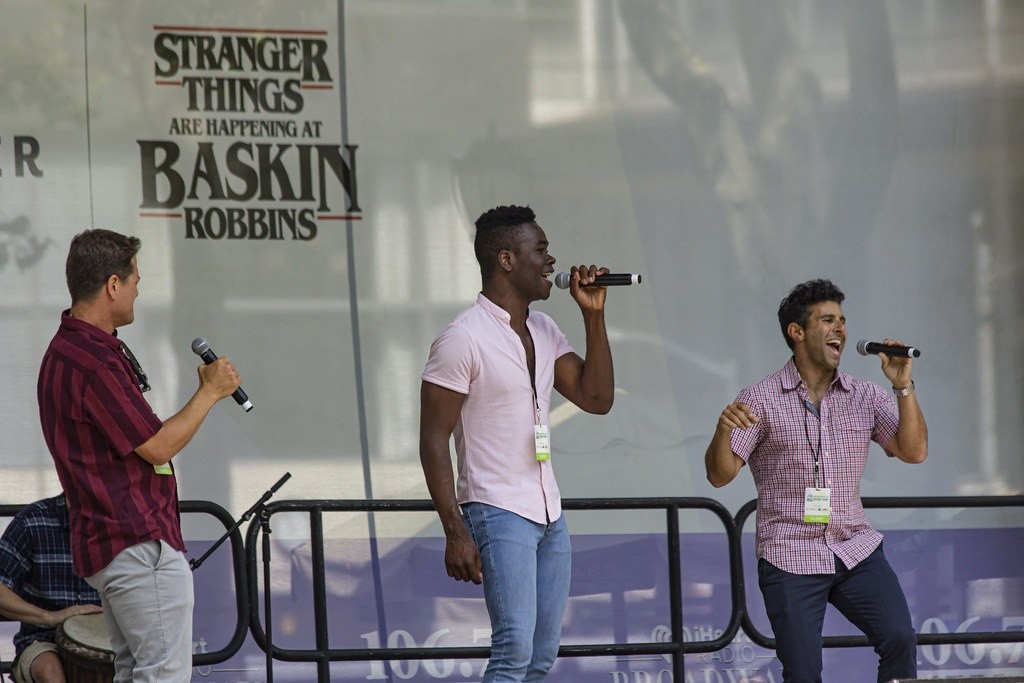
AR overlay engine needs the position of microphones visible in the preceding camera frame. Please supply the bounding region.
[555,272,642,290]
[857,339,920,359]
[191,338,254,413]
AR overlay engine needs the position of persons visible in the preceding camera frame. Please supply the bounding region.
[419,206,615,683]
[0,490,107,683]
[704,279,929,683]
[38,228,242,683]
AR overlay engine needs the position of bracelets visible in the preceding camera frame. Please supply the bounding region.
[892,379,915,397]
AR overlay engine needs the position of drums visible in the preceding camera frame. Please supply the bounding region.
[54,612,116,683]
